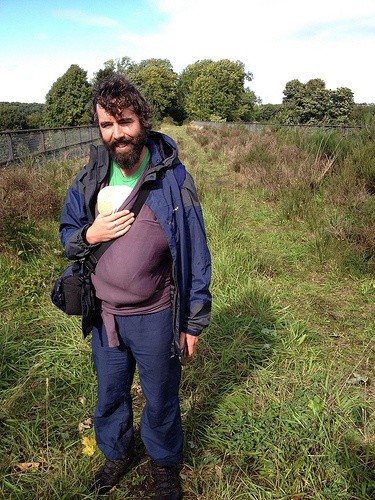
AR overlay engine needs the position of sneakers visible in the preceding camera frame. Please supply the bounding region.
[147,462,181,500]
[88,455,136,493]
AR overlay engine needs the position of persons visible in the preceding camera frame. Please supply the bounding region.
[55,76,215,500]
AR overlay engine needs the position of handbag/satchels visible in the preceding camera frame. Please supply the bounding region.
[48,260,84,314]
